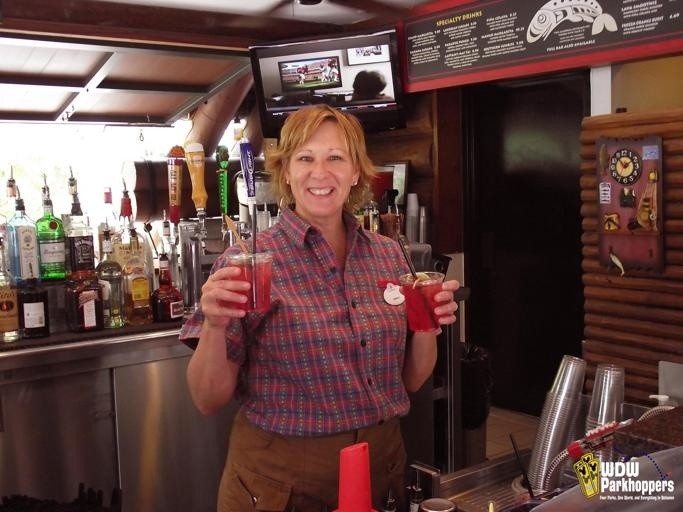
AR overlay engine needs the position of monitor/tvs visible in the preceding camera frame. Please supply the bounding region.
[248,26,407,139]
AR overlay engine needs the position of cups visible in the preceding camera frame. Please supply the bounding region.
[399,273,446,334]
[224,252,273,312]
[404,192,428,243]
[526,356,627,493]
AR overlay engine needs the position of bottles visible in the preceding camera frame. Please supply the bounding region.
[0,167,189,343]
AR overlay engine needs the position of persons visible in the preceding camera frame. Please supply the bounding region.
[316,58,339,83]
[173,102,463,512]
[295,65,308,85]
